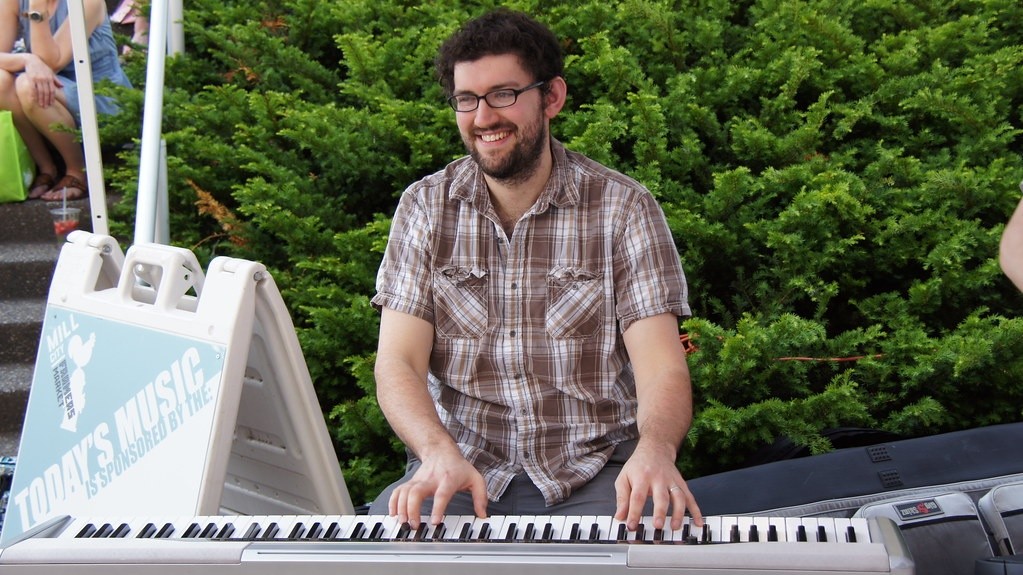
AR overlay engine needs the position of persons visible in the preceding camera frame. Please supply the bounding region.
[367,11,706,531]
[121,0,149,55]
[0,0,134,200]
[999,197,1022,292]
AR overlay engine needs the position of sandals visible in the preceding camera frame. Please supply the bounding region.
[40,175,88,201]
[28,173,61,199]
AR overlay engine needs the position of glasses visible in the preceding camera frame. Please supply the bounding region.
[448,79,545,112]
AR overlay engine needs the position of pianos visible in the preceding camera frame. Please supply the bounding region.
[0,509,922,575]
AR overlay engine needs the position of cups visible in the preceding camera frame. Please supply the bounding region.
[49,207,82,251]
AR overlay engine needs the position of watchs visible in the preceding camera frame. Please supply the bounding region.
[28,10,50,23]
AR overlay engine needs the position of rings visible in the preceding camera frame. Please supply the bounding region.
[669,487,678,491]
